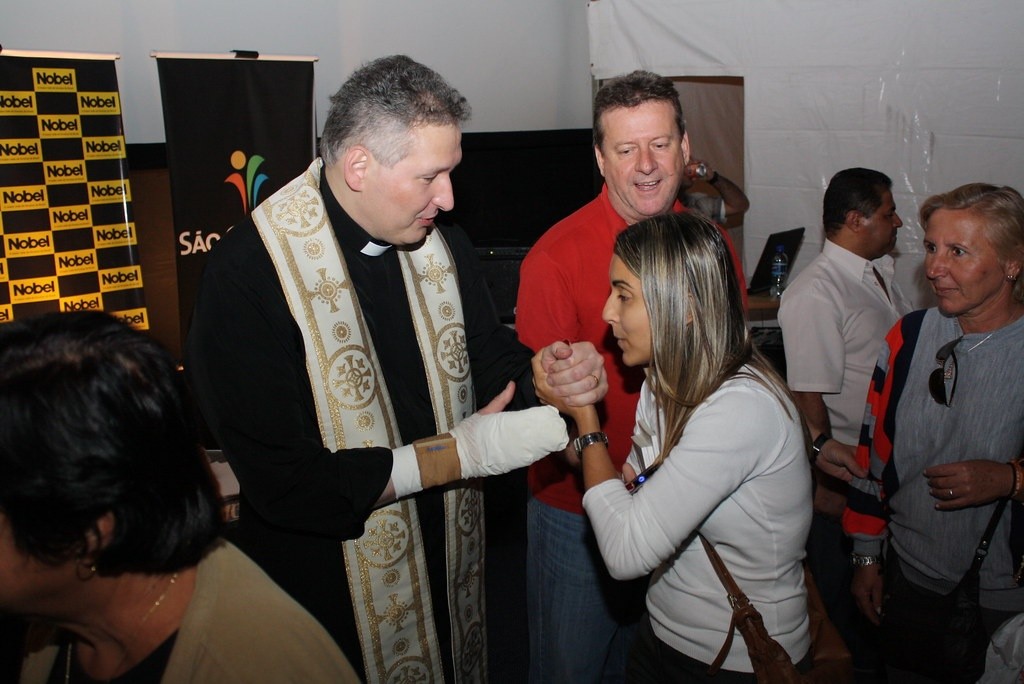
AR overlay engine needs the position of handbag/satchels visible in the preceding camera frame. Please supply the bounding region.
[736,576,851,684]
[838,541,985,684]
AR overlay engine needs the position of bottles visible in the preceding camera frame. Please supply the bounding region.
[770,245,788,297]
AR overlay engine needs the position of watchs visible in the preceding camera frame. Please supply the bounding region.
[574,432,608,458]
[848,553,881,568]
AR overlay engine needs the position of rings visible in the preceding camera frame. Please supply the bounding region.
[590,375,599,384]
[949,489,952,497]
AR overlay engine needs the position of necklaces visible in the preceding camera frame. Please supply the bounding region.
[944,302,1019,379]
[65,573,178,684]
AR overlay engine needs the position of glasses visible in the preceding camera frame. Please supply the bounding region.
[929,337,961,407]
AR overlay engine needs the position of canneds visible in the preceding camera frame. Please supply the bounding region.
[684,164,707,177]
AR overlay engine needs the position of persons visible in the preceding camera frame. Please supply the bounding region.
[187,55,609,684]
[841,183,1024,684]
[513,72,748,684]
[677,158,749,225]
[530,211,816,684]
[0,310,361,684]
[777,168,914,674]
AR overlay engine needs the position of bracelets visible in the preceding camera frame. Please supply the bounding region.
[812,432,832,460]
[707,171,719,185]
[1007,457,1022,498]
